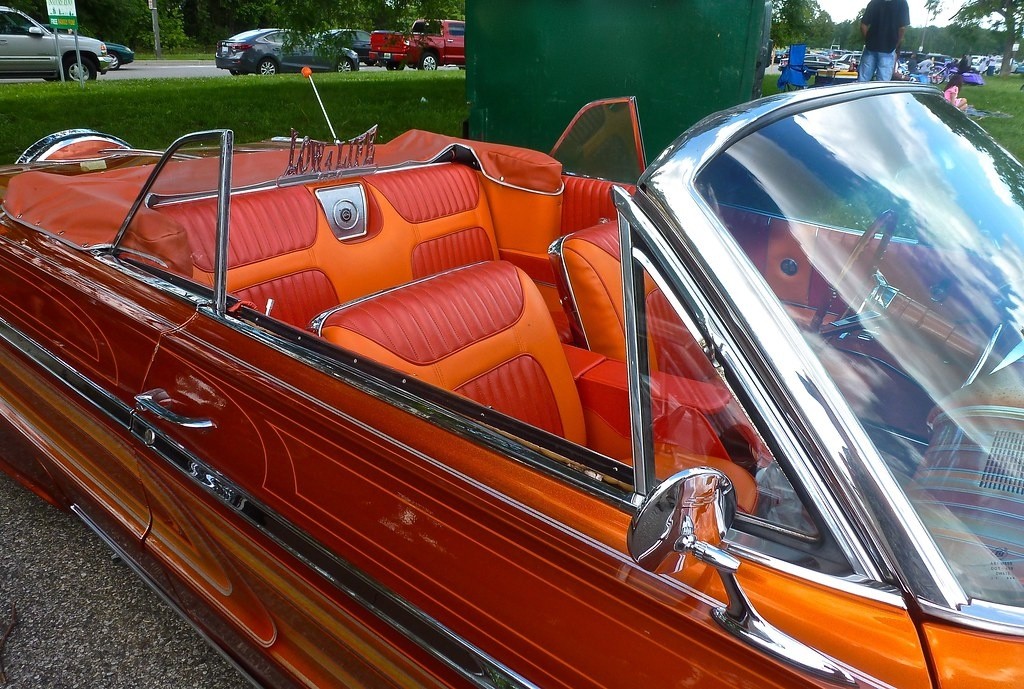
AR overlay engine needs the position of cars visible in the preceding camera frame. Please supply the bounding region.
[774,44,1023,78]
[215,29,359,76]
[0,6,114,82]
[312,28,377,67]
[41,24,135,71]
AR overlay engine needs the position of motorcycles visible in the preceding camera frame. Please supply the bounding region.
[930,59,985,85]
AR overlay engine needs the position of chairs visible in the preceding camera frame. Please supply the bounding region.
[548,215,729,390]
[308,258,761,517]
[777,43,840,93]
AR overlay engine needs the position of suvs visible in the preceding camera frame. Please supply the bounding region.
[0,79,1024,688]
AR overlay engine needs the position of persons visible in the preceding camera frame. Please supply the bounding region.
[917,57,934,83]
[857,0,910,82]
[908,54,919,76]
[956,54,973,74]
[945,74,968,110]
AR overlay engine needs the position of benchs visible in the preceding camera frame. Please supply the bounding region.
[158,163,574,381]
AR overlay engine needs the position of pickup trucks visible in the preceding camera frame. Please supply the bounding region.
[369,20,465,71]
